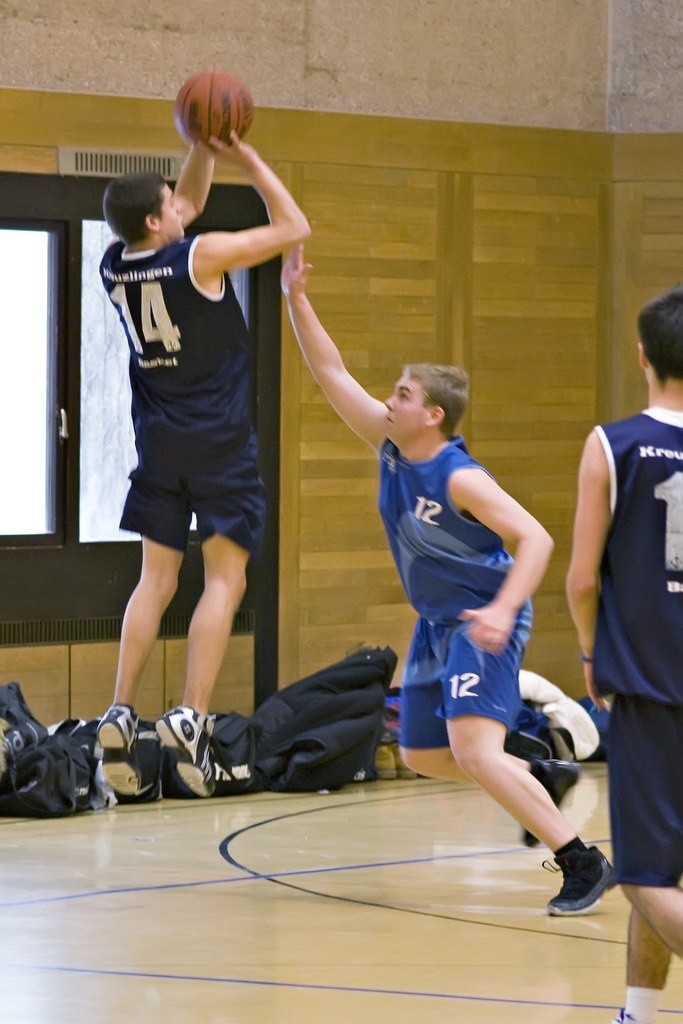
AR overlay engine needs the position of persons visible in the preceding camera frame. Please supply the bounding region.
[96,126,312,797]
[282,242,618,917]
[564,286,683,1024]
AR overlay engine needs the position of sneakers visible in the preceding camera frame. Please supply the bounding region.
[156,704,218,798]
[541,843,618,916]
[523,757,584,848]
[97,705,141,796]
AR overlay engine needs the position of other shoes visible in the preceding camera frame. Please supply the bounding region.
[376,744,396,782]
[393,740,417,779]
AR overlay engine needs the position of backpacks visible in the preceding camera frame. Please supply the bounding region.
[211,711,257,795]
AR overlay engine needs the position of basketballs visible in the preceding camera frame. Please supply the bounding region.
[173,70,252,150]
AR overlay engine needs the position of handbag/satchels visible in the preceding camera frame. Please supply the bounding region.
[0,679,92,827]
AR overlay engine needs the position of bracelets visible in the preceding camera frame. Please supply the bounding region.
[581,655,594,664]
